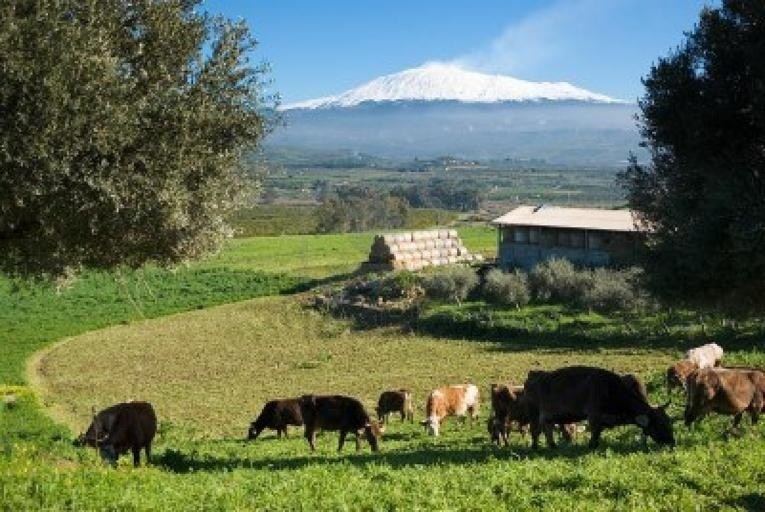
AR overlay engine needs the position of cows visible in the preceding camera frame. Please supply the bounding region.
[247,397,300,444]
[374,388,415,427]
[665,361,697,401]
[684,366,764,435]
[419,383,481,438]
[485,365,675,459]
[296,393,379,456]
[683,342,724,370]
[71,398,158,468]
